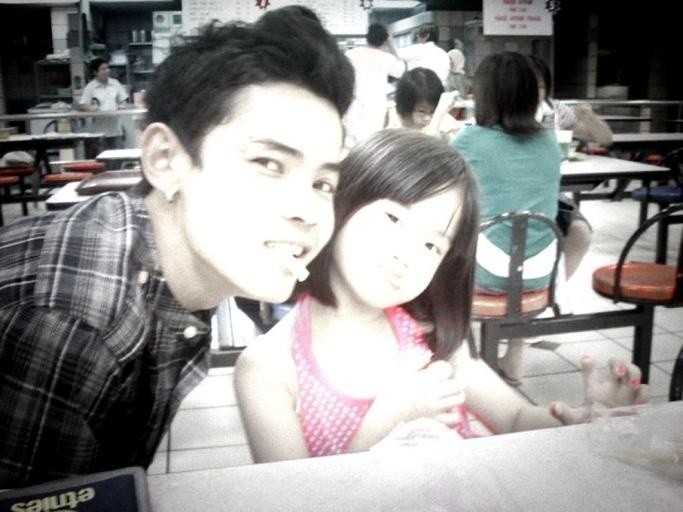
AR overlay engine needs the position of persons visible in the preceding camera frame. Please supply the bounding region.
[342,23,407,122]
[448,51,562,385]
[447,36,468,100]
[0,20,344,499]
[79,57,129,161]
[525,52,614,284]
[345,66,458,147]
[388,19,451,84]
[228,125,651,462]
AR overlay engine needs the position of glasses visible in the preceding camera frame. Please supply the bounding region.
[413,107,433,117]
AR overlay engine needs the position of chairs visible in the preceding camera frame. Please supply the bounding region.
[469,211,563,369]
[593,203,683,384]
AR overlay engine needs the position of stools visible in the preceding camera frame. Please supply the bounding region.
[64,163,106,174]
[647,155,665,165]
[0,176,19,226]
[632,187,683,264]
[0,167,34,216]
[581,147,608,187]
[44,172,93,185]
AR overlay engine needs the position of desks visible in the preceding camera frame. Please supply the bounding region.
[561,153,670,209]
[45,181,94,210]
[0,132,105,180]
[145,401,683,512]
[612,133,683,188]
[95,149,141,161]
